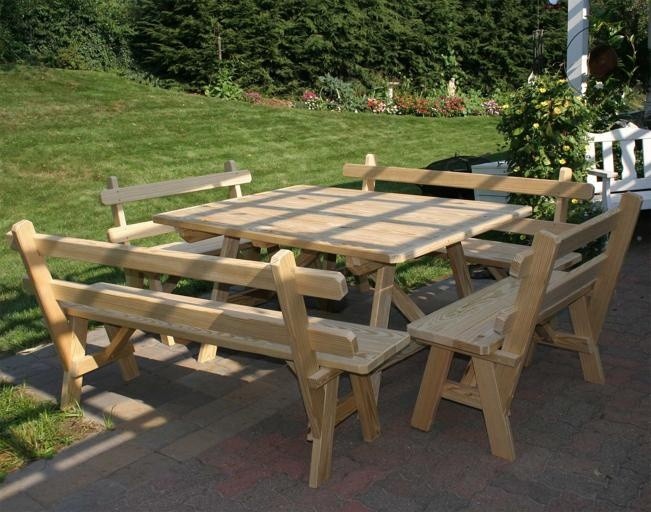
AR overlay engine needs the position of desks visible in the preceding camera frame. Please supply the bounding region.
[152,184,533,421]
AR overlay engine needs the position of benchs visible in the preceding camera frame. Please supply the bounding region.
[342,154,594,293]
[100,160,321,347]
[583,123,651,240]
[7,220,410,488]
[407,192,643,463]
